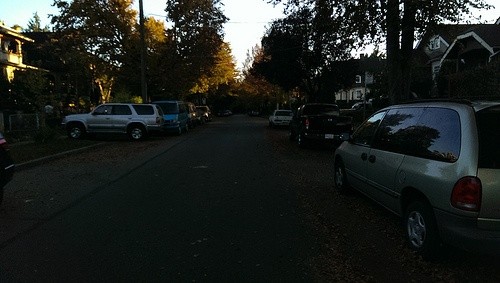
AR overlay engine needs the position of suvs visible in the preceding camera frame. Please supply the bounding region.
[60,101,165,142]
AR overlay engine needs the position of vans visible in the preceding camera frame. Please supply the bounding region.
[153,100,189,136]
[176,102,197,134]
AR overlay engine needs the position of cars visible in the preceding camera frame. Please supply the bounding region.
[0,130,15,207]
[331,95,500,259]
[195,106,212,126]
[268,109,294,129]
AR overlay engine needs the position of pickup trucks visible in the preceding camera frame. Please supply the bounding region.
[339,101,373,113]
[288,102,353,149]
